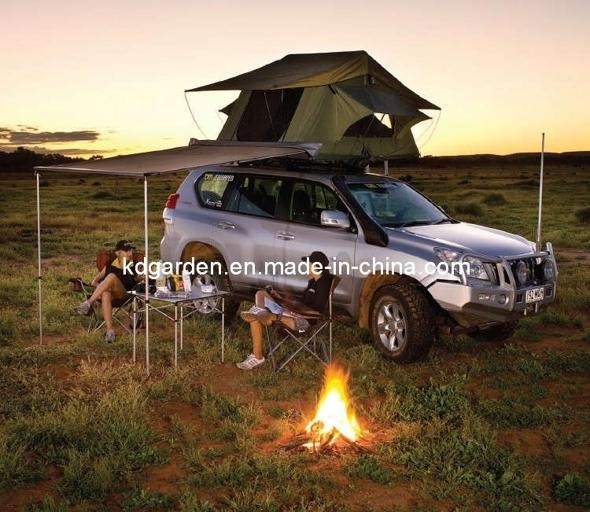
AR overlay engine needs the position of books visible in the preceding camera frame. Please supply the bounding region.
[155,291,191,298]
[264,296,292,318]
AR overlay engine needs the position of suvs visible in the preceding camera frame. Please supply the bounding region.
[159,164,557,359]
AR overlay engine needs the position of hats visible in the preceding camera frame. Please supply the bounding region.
[116,240,136,251]
[301,251,330,270]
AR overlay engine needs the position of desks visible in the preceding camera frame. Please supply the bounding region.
[126,283,234,375]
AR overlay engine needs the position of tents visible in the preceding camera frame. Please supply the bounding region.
[184,50,441,162]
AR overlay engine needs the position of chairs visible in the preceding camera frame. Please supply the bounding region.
[70,249,150,339]
[200,190,221,208]
[263,272,344,372]
[292,191,320,226]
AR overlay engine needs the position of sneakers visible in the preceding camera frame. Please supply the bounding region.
[73,301,91,316]
[235,352,266,370]
[106,329,116,343]
[240,305,269,322]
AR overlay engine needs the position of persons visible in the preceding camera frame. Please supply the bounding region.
[74,240,144,345]
[236,252,330,368]
